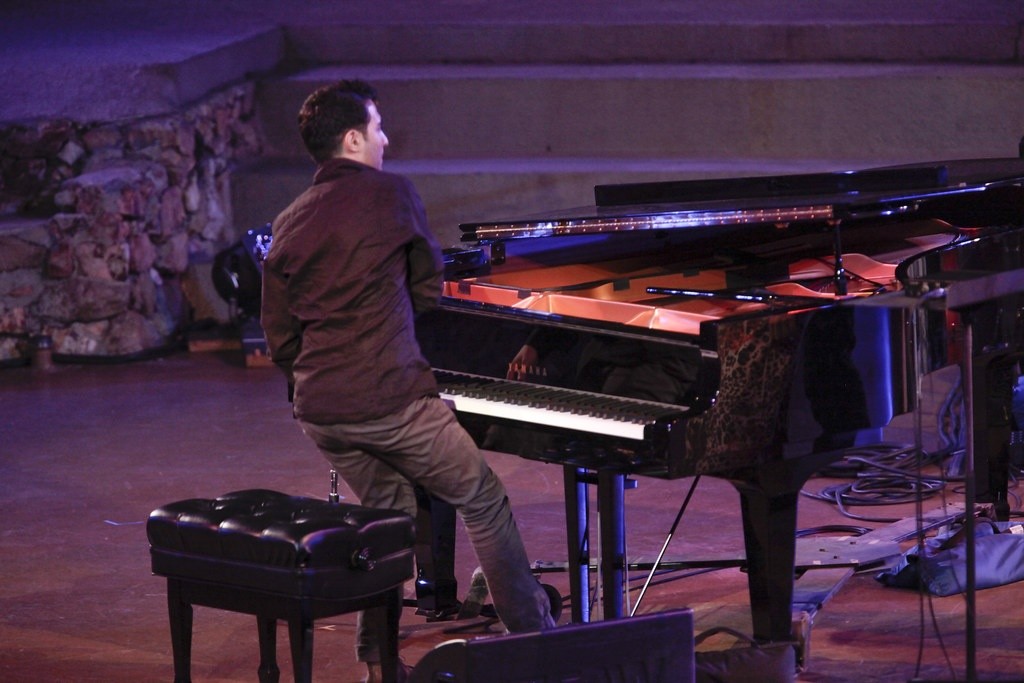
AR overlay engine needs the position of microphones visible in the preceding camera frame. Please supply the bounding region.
[828,219,849,297]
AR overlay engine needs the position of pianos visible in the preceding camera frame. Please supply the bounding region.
[288,156,1020,679]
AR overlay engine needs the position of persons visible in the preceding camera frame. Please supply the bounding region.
[263,79,565,635]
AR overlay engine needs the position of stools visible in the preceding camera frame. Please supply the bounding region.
[144,488,422,683]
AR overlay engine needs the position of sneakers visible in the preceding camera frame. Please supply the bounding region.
[542,584,563,622]
